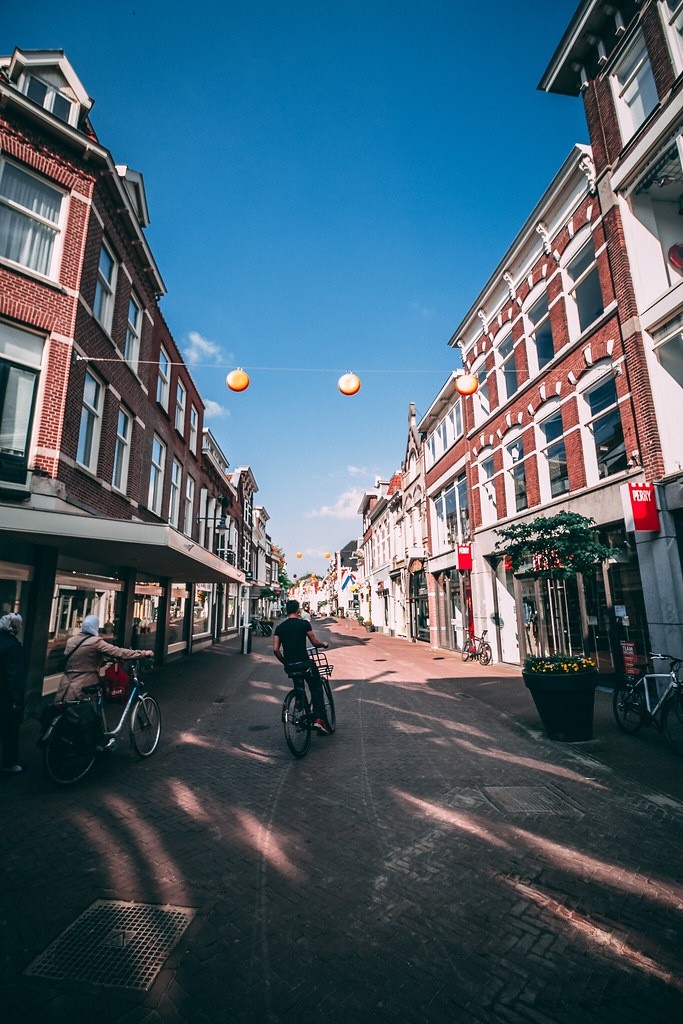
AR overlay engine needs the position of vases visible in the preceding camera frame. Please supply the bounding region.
[522,669,598,741]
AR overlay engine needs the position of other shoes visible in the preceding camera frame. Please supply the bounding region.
[2,765,21,772]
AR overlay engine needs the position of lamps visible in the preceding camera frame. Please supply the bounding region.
[196,517,229,535]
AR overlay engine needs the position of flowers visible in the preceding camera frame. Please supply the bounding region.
[523,652,596,674]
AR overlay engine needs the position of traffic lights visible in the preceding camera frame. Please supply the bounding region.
[275,590,281,594]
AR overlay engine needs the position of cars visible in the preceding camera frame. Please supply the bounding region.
[533,609,563,636]
[112,618,150,638]
[170,601,180,610]
[193,607,206,619]
[586,604,621,633]
[240,615,262,630]
[194,601,199,606]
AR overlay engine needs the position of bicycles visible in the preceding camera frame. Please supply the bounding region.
[281,645,336,758]
[254,619,272,637]
[461,630,492,666]
[36,655,161,786]
[612,652,683,758]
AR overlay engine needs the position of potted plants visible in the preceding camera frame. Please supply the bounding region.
[357,616,364,626]
[365,621,372,632]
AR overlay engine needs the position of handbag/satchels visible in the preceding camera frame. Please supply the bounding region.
[38,701,108,745]
[105,662,129,698]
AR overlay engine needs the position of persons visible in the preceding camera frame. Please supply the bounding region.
[273,600,330,735]
[54,615,154,751]
[0,613,24,772]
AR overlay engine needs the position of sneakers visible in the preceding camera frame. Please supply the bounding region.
[314,718,331,734]
